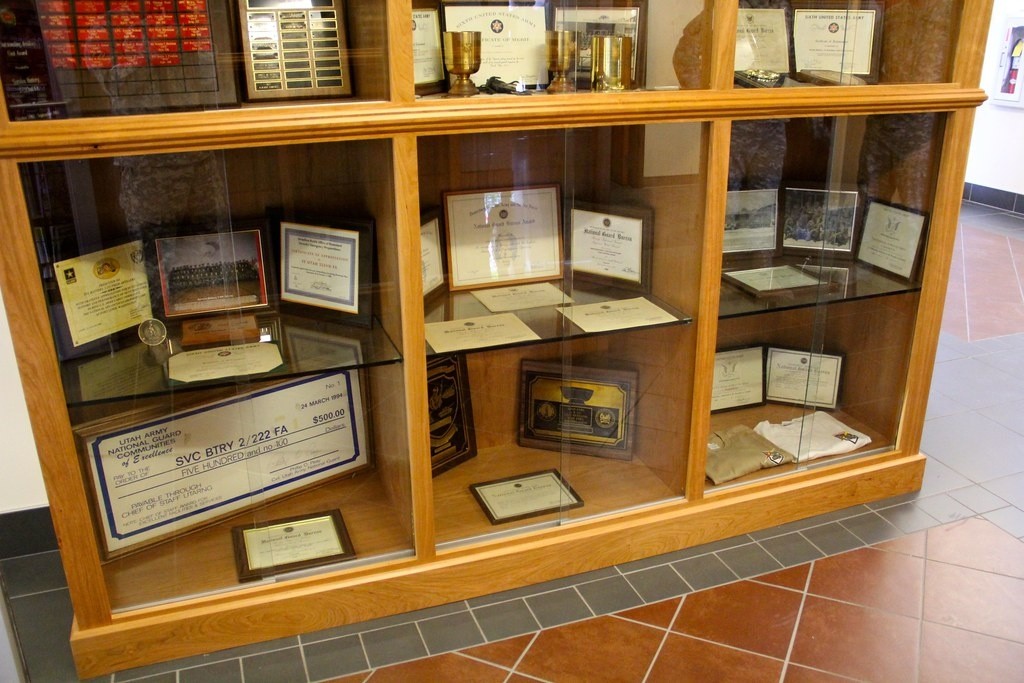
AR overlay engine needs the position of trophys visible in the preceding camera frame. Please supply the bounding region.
[546,30,581,93]
[590,35,633,92]
[442,31,481,97]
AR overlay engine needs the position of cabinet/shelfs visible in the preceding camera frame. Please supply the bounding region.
[0,0,994,678]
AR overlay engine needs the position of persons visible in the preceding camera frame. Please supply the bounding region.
[170,259,258,289]
[784,202,847,247]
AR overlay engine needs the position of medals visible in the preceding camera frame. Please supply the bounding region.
[138,319,167,345]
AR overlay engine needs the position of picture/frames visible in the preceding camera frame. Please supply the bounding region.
[781,180,869,261]
[148,222,274,324]
[722,181,785,263]
